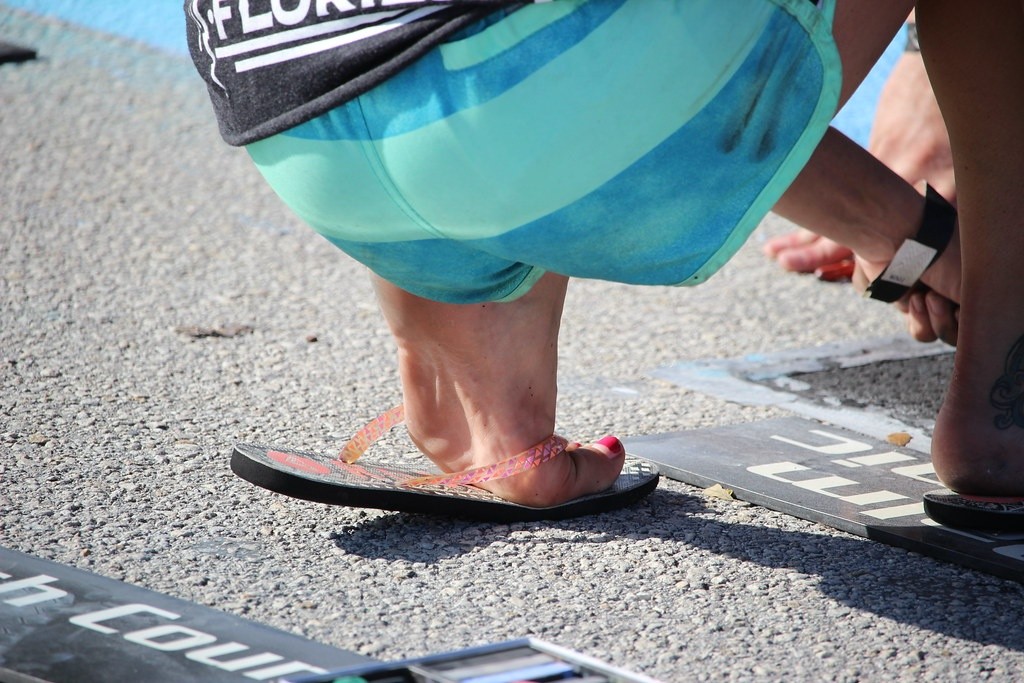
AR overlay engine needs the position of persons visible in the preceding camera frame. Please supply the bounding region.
[764,1,957,284]
[178,1,1024,531]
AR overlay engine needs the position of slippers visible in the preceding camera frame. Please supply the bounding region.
[231,398,658,521]
[923,494,1024,533]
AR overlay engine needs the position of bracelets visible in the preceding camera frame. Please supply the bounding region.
[862,180,958,304]
[903,21,920,51]
